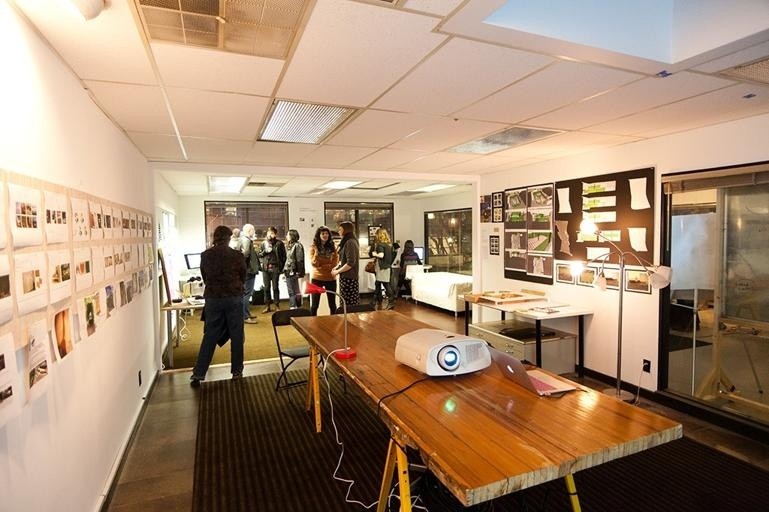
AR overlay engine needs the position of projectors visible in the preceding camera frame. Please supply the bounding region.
[395,328,491,378]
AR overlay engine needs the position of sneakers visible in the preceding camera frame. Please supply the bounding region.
[384,303,395,310]
[232,373,243,381]
[190,380,200,388]
[244,318,257,324]
[248,314,257,318]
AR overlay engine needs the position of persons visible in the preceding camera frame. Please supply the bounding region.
[283,229,306,310]
[228,228,240,254]
[393,239,423,300]
[369,227,396,312]
[257,227,287,315]
[309,224,339,317]
[237,222,261,326]
[188,224,248,388]
[330,220,361,307]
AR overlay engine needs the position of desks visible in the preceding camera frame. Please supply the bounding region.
[457,294,594,378]
[289,309,683,511]
[160,297,206,367]
[367,264,433,298]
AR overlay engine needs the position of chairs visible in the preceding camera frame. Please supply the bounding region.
[271,308,333,405]
[401,264,424,304]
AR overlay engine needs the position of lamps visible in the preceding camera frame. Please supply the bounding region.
[302,280,358,359]
[569,220,672,403]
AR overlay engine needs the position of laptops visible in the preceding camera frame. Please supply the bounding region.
[486,345,576,397]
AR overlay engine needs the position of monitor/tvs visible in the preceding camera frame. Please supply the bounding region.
[185,253,201,269]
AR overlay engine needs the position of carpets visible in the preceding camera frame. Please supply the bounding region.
[162,297,310,370]
[191,365,769,511]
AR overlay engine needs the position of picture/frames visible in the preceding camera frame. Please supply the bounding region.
[492,192,504,223]
[555,263,652,295]
[489,236,499,255]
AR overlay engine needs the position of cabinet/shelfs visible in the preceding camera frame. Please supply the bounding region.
[467,318,578,375]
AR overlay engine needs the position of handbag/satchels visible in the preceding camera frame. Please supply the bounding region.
[364,258,377,273]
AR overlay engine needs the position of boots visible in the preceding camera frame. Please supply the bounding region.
[263,303,270,313]
[274,304,280,312]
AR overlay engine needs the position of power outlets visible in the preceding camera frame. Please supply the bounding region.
[642,359,651,373]
